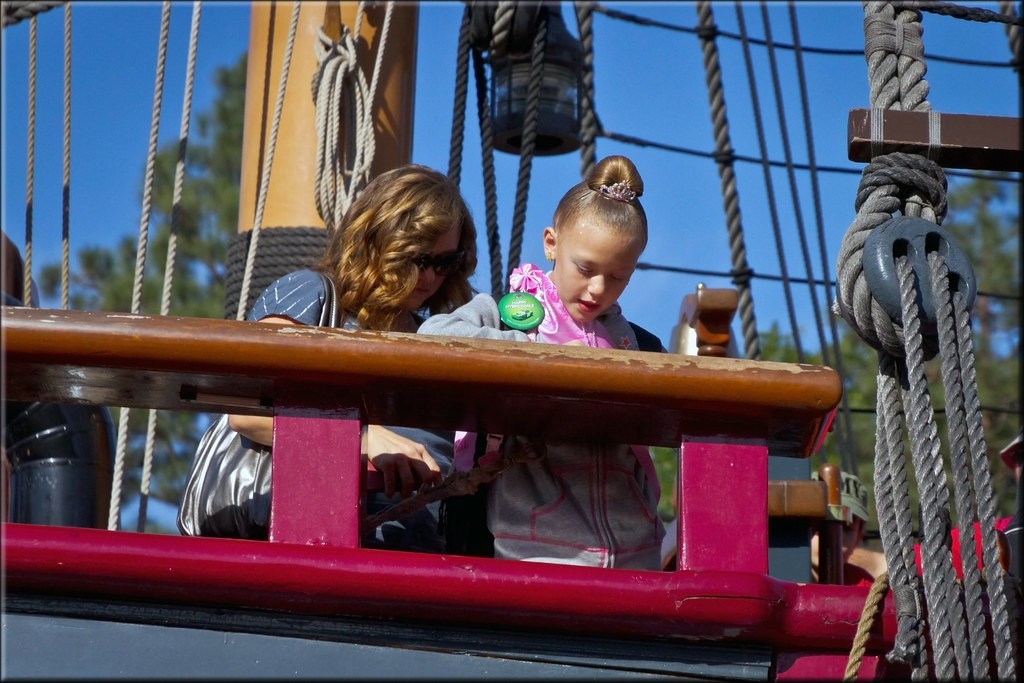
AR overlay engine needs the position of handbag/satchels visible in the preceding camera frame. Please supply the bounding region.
[176,273,338,542]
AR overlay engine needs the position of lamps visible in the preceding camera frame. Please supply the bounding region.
[490,0,584,158]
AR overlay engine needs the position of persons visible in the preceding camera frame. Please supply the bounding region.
[176,164,478,556]
[416,155,668,572]
[811,471,882,587]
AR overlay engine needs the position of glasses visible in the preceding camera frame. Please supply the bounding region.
[410,250,465,277]
